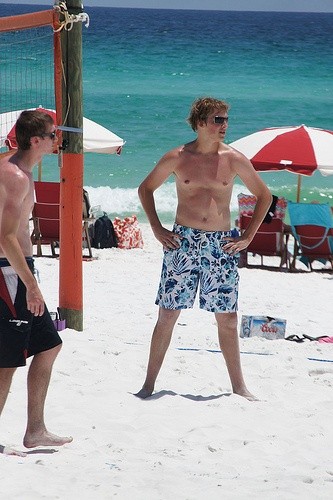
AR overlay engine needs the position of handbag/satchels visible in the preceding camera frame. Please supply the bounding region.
[112,215,145,249]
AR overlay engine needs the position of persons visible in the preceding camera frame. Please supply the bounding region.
[133,96,273,402]
[0,110,73,457]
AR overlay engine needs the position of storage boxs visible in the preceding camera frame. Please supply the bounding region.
[239,315,287,340]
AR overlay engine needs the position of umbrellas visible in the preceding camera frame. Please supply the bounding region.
[0,103,127,156]
[228,121,332,203]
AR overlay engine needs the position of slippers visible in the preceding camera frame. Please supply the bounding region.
[303,334,330,341]
[284,334,305,343]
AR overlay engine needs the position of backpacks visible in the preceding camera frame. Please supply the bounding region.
[93,211,117,249]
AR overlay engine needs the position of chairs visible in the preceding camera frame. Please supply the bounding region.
[234,193,291,271]
[29,181,93,259]
[287,201,333,273]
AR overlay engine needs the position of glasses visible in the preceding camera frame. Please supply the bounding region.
[35,128,56,138]
[206,116,228,124]
